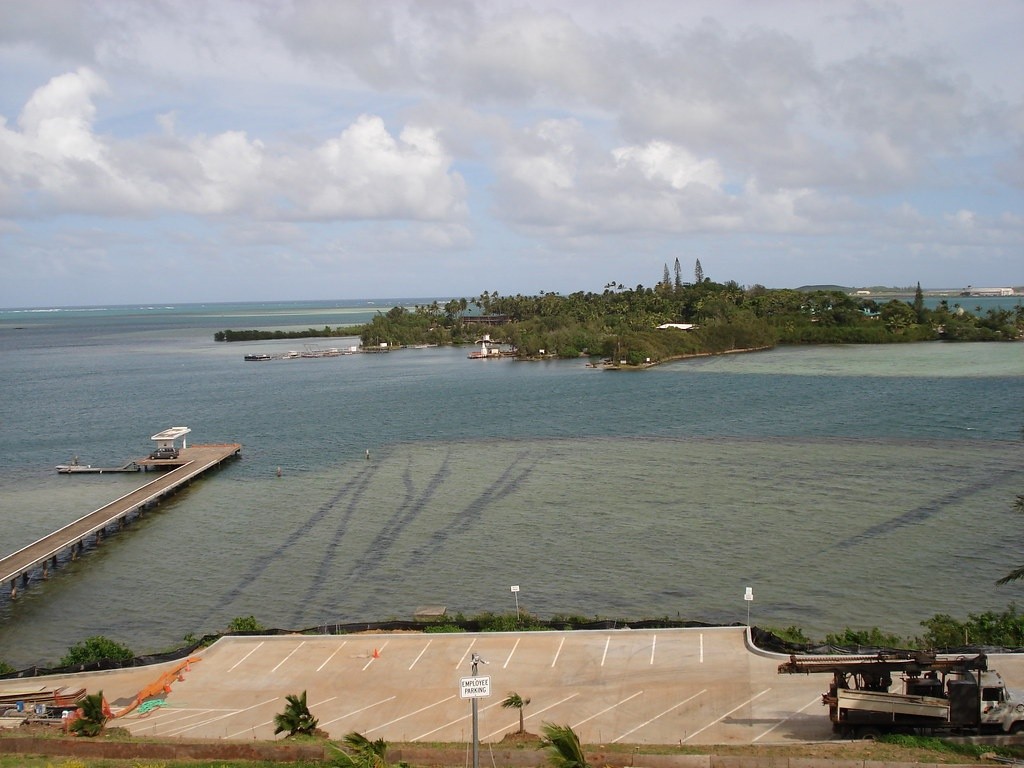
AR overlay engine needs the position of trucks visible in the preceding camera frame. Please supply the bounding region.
[829,666,1024,741]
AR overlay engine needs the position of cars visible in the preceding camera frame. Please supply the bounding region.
[149,447,180,460]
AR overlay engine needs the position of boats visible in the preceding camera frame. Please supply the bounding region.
[244,353,271,362]
